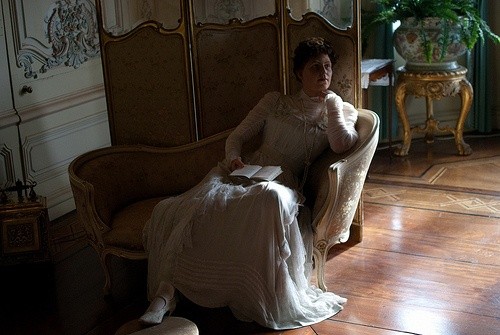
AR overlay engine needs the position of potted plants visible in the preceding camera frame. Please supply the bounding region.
[362,0,500,73]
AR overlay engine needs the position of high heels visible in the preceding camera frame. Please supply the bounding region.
[140,293,176,324]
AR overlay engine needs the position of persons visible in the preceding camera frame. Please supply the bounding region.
[140,36,358,330]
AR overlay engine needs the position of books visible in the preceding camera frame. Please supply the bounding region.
[228,164,284,181]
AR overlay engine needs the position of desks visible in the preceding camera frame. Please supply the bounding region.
[393,66,474,157]
[361,57,395,179]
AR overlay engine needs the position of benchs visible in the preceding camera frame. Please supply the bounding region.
[68,109,380,296]
